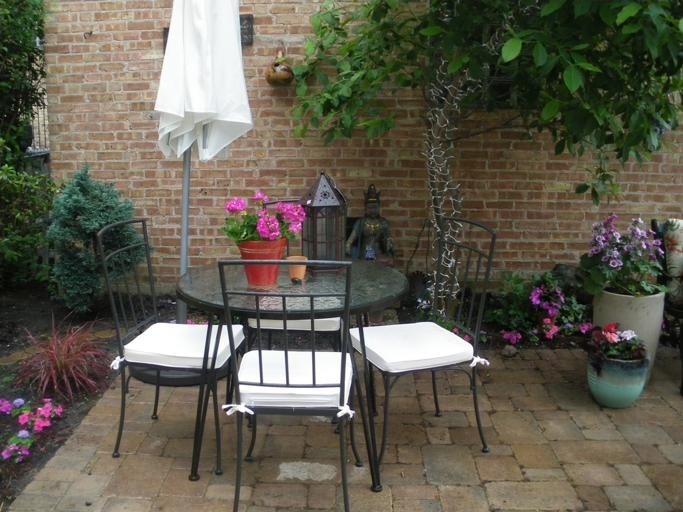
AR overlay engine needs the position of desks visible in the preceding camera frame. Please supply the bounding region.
[176,258,409,491]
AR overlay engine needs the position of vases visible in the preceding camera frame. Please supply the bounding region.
[587,354,650,409]
[237,238,286,285]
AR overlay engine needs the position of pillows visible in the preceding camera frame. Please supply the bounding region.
[661,216,682,301]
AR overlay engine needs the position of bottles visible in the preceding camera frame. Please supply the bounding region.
[265,47,293,86]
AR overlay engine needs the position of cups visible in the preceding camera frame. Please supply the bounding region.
[286,256,309,282]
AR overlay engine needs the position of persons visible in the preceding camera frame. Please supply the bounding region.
[345,201,394,261]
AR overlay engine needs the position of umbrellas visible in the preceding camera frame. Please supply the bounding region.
[153,0,254,276]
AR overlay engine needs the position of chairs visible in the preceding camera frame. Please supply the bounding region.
[96,217,244,482]
[222,200,368,417]
[650,218,682,397]
[215,260,362,511]
[350,214,496,467]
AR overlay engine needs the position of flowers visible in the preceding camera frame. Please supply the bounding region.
[577,215,668,375]
[223,185,306,242]
